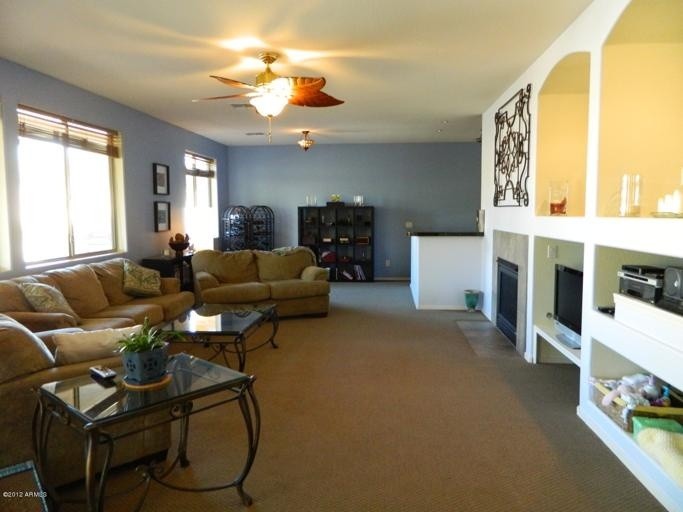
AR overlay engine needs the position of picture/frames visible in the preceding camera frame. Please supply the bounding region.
[154,201,171,232]
[153,162,170,194]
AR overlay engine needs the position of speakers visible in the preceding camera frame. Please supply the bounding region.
[661,266,683,302]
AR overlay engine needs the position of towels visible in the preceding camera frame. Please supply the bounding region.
[637,428,683,490]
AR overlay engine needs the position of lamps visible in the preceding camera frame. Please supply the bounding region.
[297,130,313,150]
[247,84,290,120]
[191,48,345,122]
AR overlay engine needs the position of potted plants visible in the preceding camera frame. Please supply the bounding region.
[116,318,182,385]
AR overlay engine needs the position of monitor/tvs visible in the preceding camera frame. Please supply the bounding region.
[552,263,583,349]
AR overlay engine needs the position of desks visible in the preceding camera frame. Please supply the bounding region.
[146,250,194,290]
[35,353,264,511]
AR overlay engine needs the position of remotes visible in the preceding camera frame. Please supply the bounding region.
[89,364,117,379]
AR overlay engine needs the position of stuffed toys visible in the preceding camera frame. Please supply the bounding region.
[596,366,672,424]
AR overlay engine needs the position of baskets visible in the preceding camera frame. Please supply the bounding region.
[592,378,683,431]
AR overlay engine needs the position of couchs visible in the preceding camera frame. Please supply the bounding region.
[192,248,331,318]
[1,313,170,493]
[0,258,196,332]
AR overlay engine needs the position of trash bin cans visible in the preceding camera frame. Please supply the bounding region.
[465,288,479,314]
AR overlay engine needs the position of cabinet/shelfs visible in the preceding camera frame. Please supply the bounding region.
[297,206,373,283]
[224,204,274,250]
[586,239,683,509]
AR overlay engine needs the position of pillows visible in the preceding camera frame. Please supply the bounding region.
[124,260,163,296]
[21,283,80,321]
[48,326,145,364]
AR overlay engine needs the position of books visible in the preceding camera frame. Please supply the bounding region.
[336,263,368,282]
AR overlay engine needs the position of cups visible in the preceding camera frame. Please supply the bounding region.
[549,179,567,215]
[619,174,640,216]
